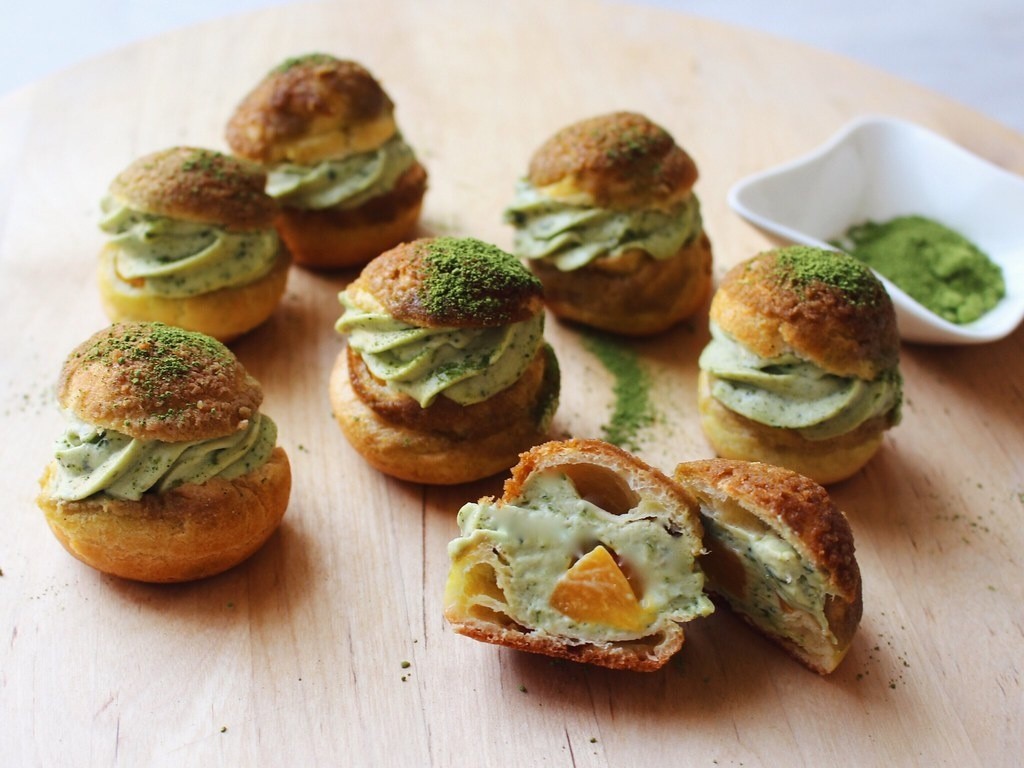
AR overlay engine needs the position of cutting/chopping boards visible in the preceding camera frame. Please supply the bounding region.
[3,0,1023,768]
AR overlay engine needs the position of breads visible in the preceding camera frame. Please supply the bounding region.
[672,457,863,677]
[98,143,293,342]
[225,53,428,270]
[497,110,714,336]
[326,233,561,484]
[33,321,293,583]
[444,436,709,672]
[691,244,903,481]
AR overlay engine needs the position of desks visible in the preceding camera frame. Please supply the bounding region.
[1,0,1023,768]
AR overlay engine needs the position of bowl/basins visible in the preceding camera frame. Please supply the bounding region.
[725,112,1024,344]
